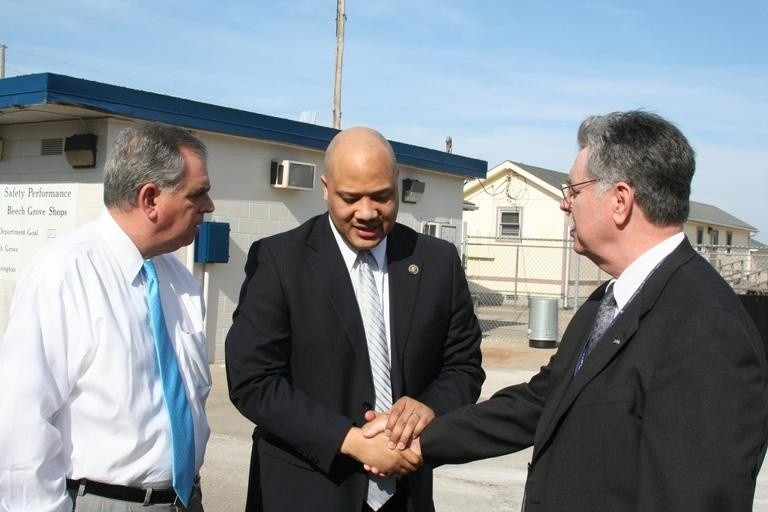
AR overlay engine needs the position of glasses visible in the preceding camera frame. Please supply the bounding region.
[560,179,598,203]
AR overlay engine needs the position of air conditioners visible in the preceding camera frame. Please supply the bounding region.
[270,159,316,192]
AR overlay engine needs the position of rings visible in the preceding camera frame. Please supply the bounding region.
[411,413,421,419]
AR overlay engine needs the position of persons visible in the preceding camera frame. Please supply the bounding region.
[0,122,214,512]
[361,112,768,511]
[224,127,487,511]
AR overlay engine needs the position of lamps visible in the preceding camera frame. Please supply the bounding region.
[65,135,98,169]
[402,179,425,204]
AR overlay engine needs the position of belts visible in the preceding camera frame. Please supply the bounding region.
[68,477,200,504]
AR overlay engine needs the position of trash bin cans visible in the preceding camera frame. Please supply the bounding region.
[528,296,559,349]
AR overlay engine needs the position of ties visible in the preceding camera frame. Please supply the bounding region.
[357,252,396,511]
[143,260,194,508]
[585,282,616,358]
